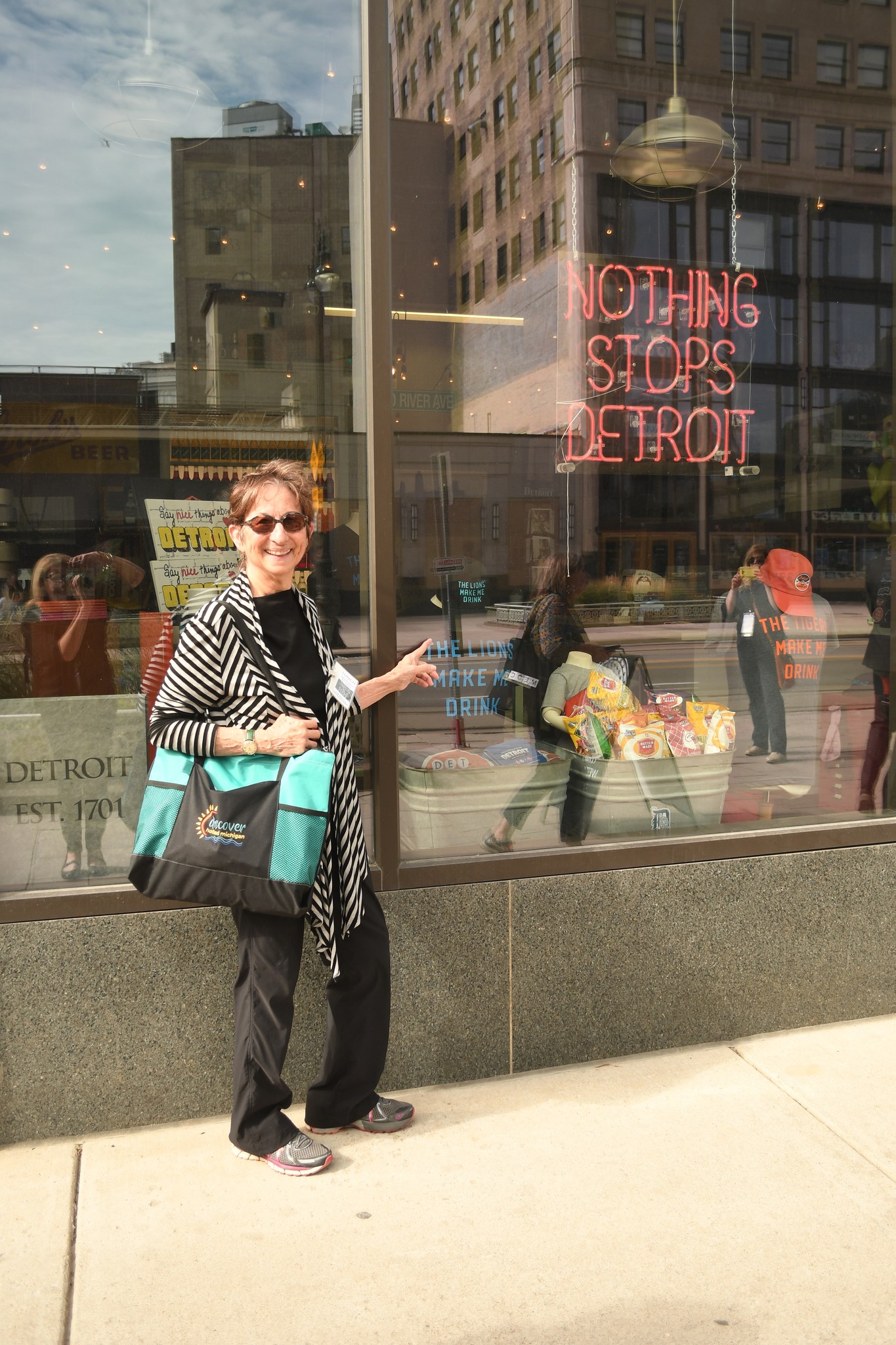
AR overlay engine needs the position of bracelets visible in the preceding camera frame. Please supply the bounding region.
[96,551,102,564]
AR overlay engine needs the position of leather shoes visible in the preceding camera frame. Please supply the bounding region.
[60,850,82,880]
[87,850,107,877]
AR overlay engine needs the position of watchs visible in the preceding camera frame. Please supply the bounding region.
[241,729,257,756]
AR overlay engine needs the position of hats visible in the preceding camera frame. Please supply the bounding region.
[758,549,816,617]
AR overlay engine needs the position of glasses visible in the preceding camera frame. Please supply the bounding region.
[44,571,80,583]
[229,512,309,535]
[747,556,765,565]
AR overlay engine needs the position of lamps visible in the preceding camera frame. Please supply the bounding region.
[73,0,224,153]
[608,0,744,203]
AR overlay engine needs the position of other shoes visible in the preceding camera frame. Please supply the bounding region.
[480,833,515,853]
[744,746,769,756]
[882,800,896,817]
[766,752,787,763]
[857,785,876,815]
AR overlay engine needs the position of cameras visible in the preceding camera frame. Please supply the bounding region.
[77,567,95,589]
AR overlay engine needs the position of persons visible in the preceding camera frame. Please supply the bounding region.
[0,574,27,622]
[858,530,896,816]
[144,459,439,1177]
[701,548,839,799]
[721,544,787,764]
[20,549,147,881]
[138,485,242,770]
[483,550,626,852]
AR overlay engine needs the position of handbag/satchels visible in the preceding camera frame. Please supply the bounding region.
[125,600,336,920]
[484,591,570,734]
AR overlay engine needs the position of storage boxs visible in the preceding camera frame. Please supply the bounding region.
[391,741,577,851]
[551,738,733,839]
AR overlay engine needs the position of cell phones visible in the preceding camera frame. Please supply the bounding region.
[604,645,621,649]
[739,566,760,577]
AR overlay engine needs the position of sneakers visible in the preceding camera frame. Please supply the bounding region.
[303,1088,415,1136]
[229,1112,333,1177]
